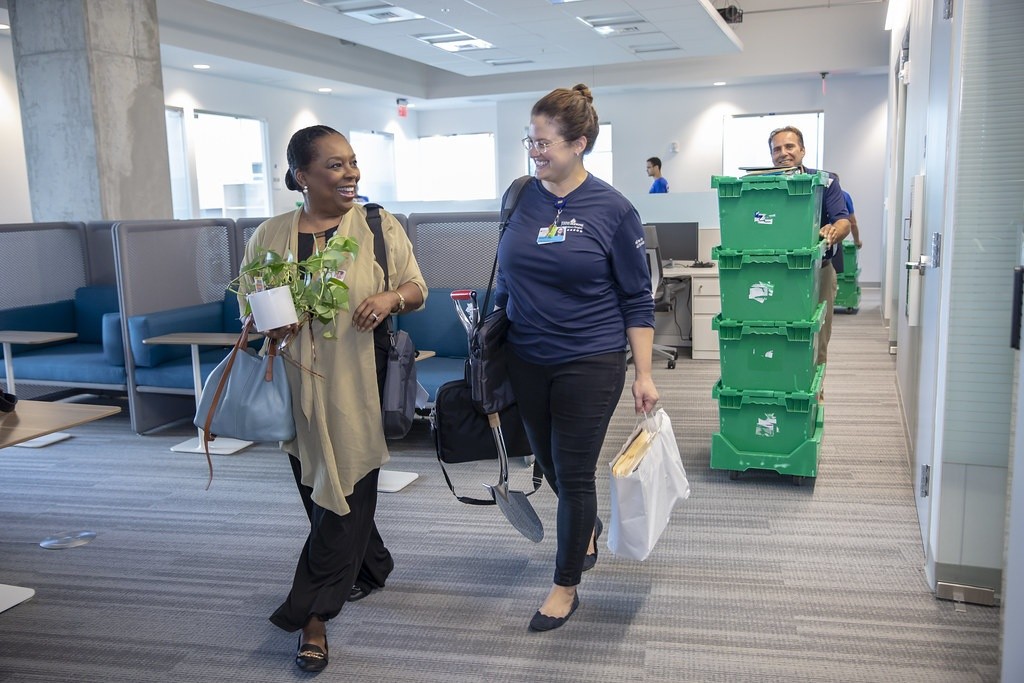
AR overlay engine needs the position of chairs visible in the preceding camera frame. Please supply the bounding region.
[623,226,677,370]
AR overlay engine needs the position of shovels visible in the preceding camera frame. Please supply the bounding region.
[451,288,545,544]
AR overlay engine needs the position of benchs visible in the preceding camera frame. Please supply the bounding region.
[128,287,263,395]
[386,289,495,408]
[0,284,127,391]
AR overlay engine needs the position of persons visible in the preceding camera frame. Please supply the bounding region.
[336,271,343,279]
[769,125,851,400]
[493,82,658,632]
[646,157,670,193]
[237,124,428,672]
[555,228,564,236]
[832,173,861,274]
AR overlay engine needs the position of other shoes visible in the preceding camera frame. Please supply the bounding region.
[581,516,603,572]
[530,587,580,630]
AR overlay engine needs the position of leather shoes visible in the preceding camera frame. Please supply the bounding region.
[348,584,372,602]
[296,633,328,672]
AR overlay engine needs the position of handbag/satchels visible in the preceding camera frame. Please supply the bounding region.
[382,329,418,440]
[429,360,543,505]
[372,312,394,411]
[606,404,690,561]
[193,311,297,443]
[468,309,517,415]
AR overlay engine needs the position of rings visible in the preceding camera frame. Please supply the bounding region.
[372,313,379,321]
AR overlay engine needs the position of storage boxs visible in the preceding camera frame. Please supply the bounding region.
[711,173,859,483]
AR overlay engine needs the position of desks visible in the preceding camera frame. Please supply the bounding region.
[144,333,264,454]
[0,400,121,613]
[662,260,722,359]
[377,350,436,492]
[0,331,77,448]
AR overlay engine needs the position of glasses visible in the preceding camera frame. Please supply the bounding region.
[522,137,577,154]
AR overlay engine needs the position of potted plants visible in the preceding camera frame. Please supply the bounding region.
[227,235,360,338]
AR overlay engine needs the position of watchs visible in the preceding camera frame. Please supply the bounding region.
[391,290,405,313]
